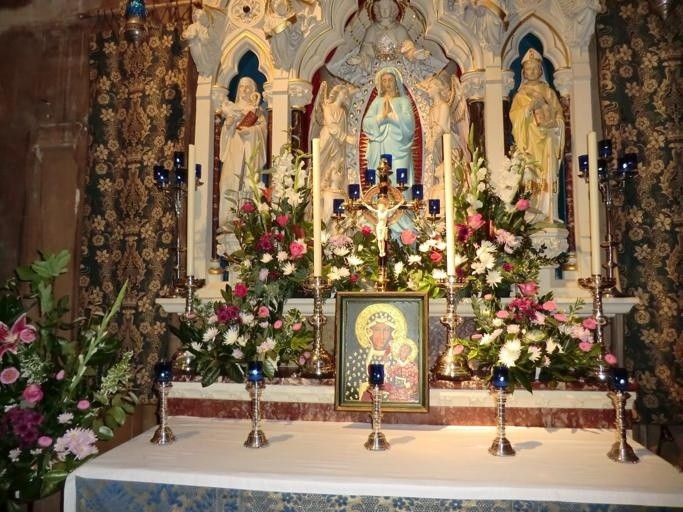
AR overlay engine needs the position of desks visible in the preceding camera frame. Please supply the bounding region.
[56,407,683,511]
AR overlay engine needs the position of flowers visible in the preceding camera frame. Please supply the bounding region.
[447,121,618,390]
[0,241,146,510]
[167,143,325,390]
[311,219,469,296]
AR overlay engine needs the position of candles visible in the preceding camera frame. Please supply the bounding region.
[492,365,509,388]
[330,149,440,216]
[151,151,204,192]
[577,137,641,178]
[244,361,264,384]
[153,360,173,384]
[611,368,629,390]
[366,364,384,386]
[310,137,323,281]
[440,133,457,286]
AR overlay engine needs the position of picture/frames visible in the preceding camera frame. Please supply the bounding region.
[330,287,432,414]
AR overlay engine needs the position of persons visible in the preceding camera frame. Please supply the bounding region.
[215,77,268,259]
[429,160,458,214]
[361,67,416,188]
[309,81,357,192]
[342,303,420,403]
[360,199,405,258]
[344,1,431,66]
[321,167,348,227]
[508,49,564,222]
[414,75,469,187]
